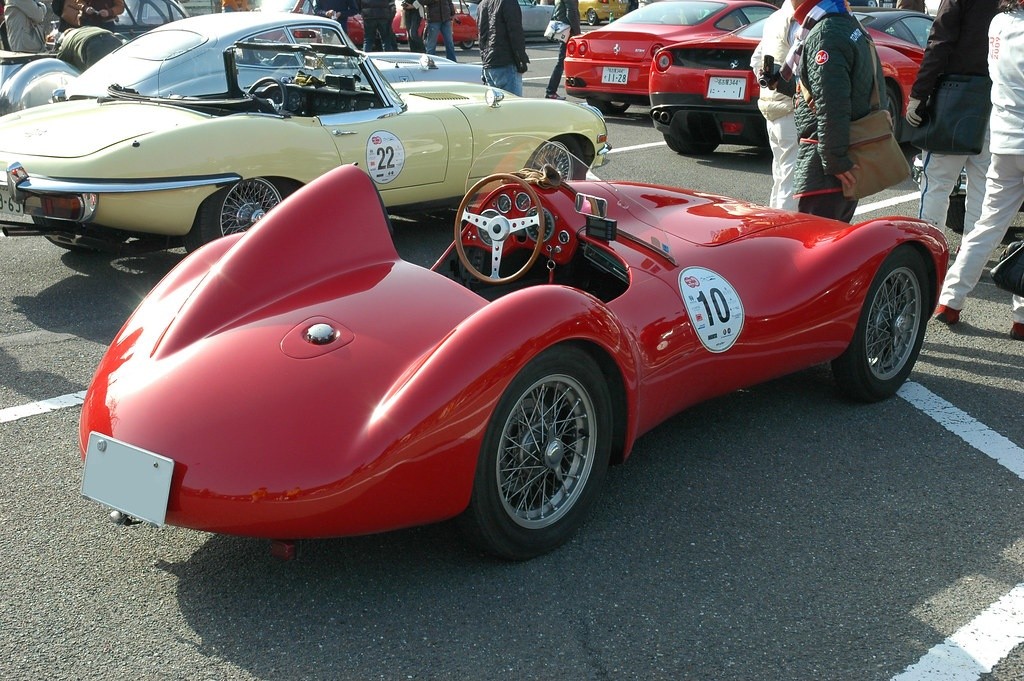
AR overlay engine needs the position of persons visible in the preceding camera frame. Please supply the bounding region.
[221,0,252,12]
[545,0,581,100]
[0,0,125,53]
[906,0,999,253]
[790,0,889,224]
[477,0,531,97]
[314,0,457,63]
[896,0,925,14]
[750,0,803,212]
[936,0,1024,340]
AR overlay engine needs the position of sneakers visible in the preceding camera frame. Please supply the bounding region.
[1010,323,1024,340]
[935,304,959,324]
[546,92,566,100]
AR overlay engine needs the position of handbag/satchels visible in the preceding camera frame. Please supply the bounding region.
[988,240,1024,298]
[544,18,572,44]
[911,70,992,156]
[842,111,911,203]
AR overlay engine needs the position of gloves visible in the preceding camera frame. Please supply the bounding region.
[516,62,527,74]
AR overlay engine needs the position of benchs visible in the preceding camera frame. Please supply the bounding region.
[105,82,258,118]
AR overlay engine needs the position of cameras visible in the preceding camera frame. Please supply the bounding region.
[758,55,782,88]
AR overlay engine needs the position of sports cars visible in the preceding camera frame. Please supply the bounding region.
[649,6,935,154]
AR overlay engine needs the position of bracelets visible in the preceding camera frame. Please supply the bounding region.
[107,8,114,17]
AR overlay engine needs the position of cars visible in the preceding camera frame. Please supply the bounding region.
[78,134,949,565]
[563,0,782,117]
[338,0,480,51]
[2,0,611,273]
[450,0,556,48]
[578,0,631,26]
